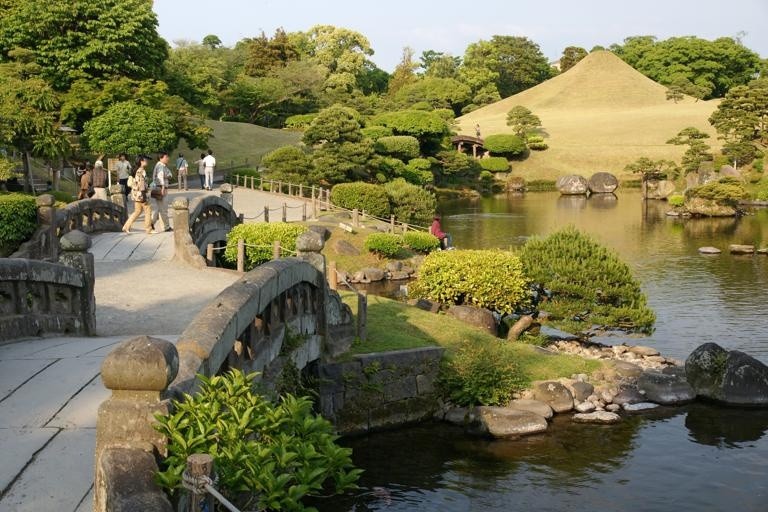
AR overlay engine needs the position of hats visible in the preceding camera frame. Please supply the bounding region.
[94,160,104,167]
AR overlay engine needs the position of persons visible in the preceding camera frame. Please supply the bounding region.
[428,213,456,250]
[177,153,188,191]
[122,156,158,234]
[475,123,481,138]
[114,153,133,202]
[203,149,216,191]
[92,160,108,201]
[150,151,174,232]
[195,153,206,188]
[80,164,94,199]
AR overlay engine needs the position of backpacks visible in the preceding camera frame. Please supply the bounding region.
[126,175,137,188]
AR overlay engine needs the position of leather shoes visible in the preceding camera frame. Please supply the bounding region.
[122,224,174,236]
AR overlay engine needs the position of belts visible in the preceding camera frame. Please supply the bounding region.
[153,185,167,189]
[95,186,106,188]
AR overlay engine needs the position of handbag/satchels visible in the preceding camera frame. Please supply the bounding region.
[130,191,146,203]
[150,188,164,200]
[87,186,95,198]
